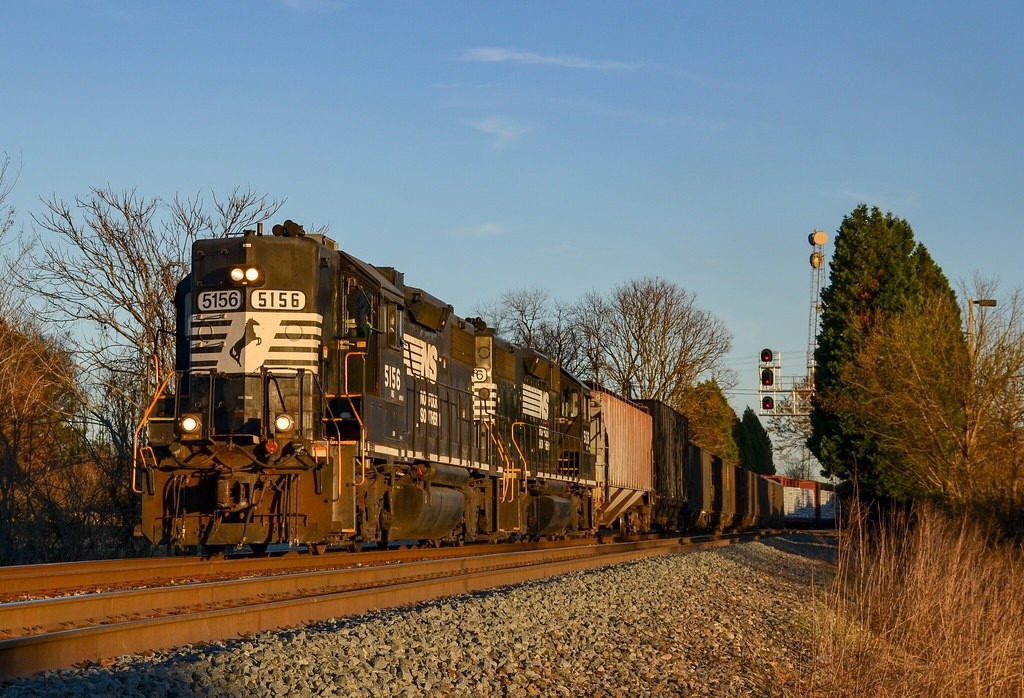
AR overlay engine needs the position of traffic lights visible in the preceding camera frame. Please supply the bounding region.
[762,349,774,409]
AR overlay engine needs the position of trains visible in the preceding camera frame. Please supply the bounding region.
[132,217,848,558]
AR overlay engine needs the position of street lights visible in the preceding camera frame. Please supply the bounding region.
[967,297,998,409]
